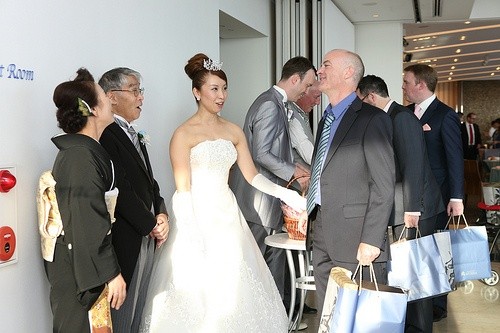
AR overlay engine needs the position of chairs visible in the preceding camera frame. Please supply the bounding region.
[461,158,498,225]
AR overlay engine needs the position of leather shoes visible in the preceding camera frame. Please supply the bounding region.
[296,303,317,314]
[289,322,308,331]
[432,311,447,322]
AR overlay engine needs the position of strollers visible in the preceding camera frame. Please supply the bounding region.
[471,201,499,286]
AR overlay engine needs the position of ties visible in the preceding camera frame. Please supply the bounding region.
[468,125,473,145]
[113,117,147,170]
[413,105,421,121]
[306,113,335,216]
[293,104,312,136]
[284,102,289,117]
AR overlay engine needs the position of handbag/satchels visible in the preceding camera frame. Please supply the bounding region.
[317,259,362,333]
[332,260,407,333]
[388,225,457,301]
[437,214,491,282]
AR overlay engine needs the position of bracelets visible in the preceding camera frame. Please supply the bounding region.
[157,214,170,222]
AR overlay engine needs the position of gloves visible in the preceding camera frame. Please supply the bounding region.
[176,192,202,260]
[251,173,307,213]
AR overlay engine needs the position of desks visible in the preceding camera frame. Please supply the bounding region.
[265,232,315,333]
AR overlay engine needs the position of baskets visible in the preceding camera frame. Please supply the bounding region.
[284,175,311,240]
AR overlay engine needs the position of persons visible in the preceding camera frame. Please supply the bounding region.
[98,67,170,333]
[36,67,126,333]
[137,53,307,333]
[355,75,446,333]
[481,116,500,154]
[461,113,481,160]
[455,112,464,124]
[402,63,464,322]
[228,56,318,331]
[285,77,322,175]
[306,49,396,333]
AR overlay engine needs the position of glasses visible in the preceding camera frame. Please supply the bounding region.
[111,87,144,97]
